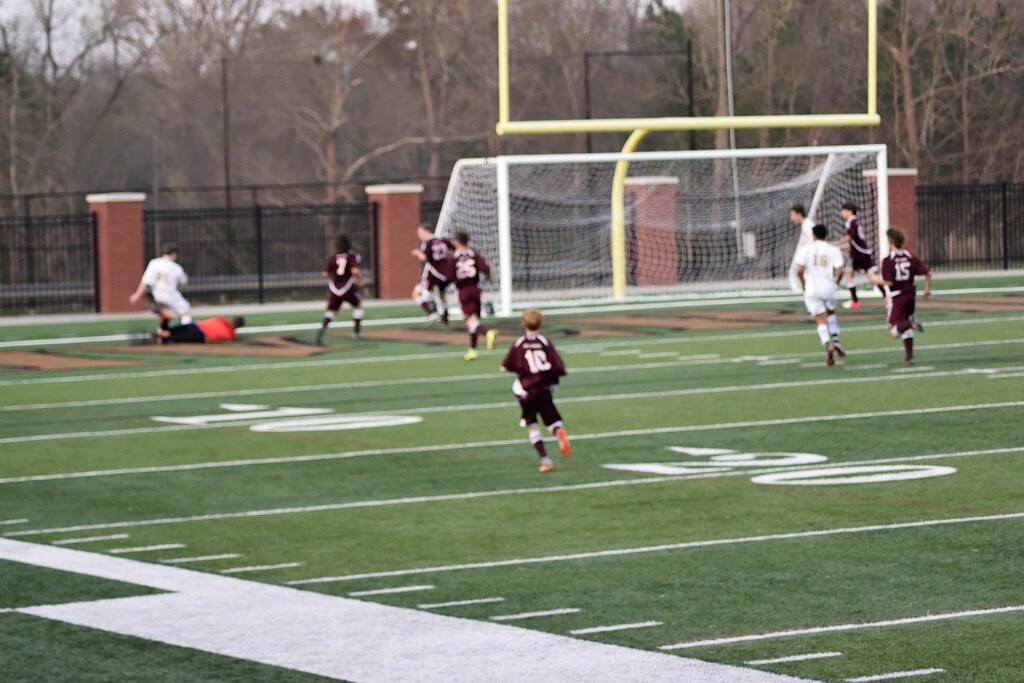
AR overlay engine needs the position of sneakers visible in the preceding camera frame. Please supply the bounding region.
[464,348,479,361]
[486,329,498,351]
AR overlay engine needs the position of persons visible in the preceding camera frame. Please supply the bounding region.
[789,202,932,367]
[129,222,499,362]
[499,310,571,473]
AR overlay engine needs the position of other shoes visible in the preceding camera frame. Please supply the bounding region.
[316,328,325,347]
[827,345,835,366]
[152,306,172,319]
[842,300,862,309]
[556,428,572,457]
[908,315,924,332]
[902,358,915,367]
[155,326,171,338]
[833,341,846,358]
[540,458,554,472]
[423,314,441,329]
[352,332,364,341]
